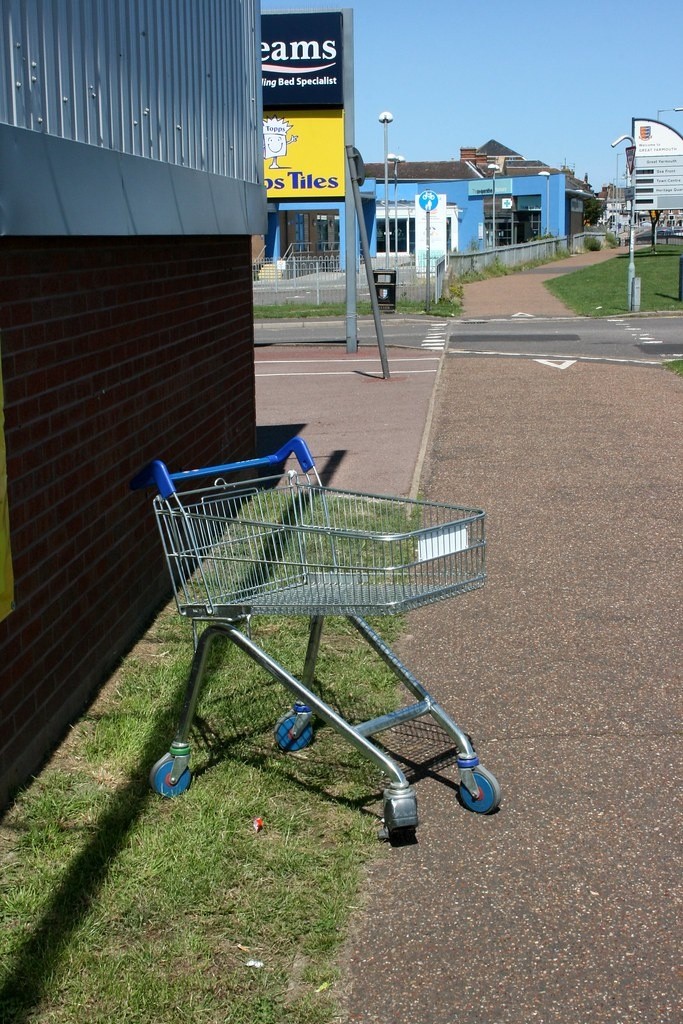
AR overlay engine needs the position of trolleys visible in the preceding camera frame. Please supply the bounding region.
[143,437,503,845]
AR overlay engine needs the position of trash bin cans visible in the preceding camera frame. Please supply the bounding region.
[371,268,397,313]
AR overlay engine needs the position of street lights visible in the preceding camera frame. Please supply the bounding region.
[488,164,500,249]
[539,171,550,238]
[387,153,405,264]
[382,111,393,268]
